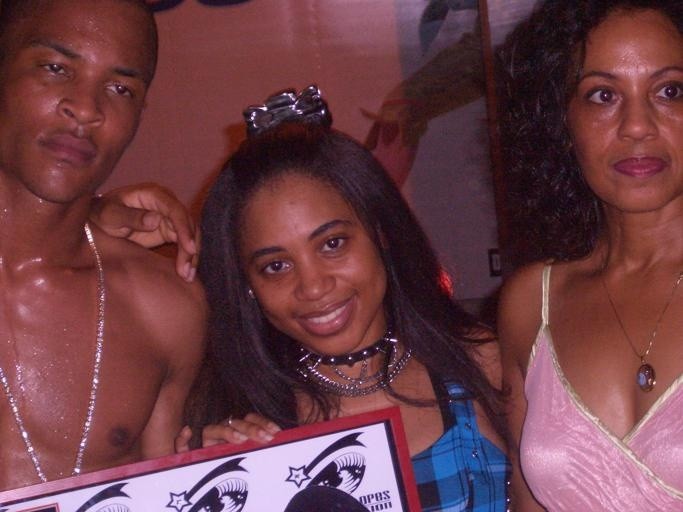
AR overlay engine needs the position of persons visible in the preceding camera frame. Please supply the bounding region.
[494,1,683,512]
[88,179,203,286]
[172,80,514,512]
[0,0,215,496]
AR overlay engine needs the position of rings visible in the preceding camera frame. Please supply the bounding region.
[222,412,232,426]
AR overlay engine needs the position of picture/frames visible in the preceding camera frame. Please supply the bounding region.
[0,406,423,512]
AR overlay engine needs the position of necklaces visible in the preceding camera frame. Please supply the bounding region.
[297,323,395,370]
[598,247,683,394]
[299,349,413,397]
[0,221,107,489]
